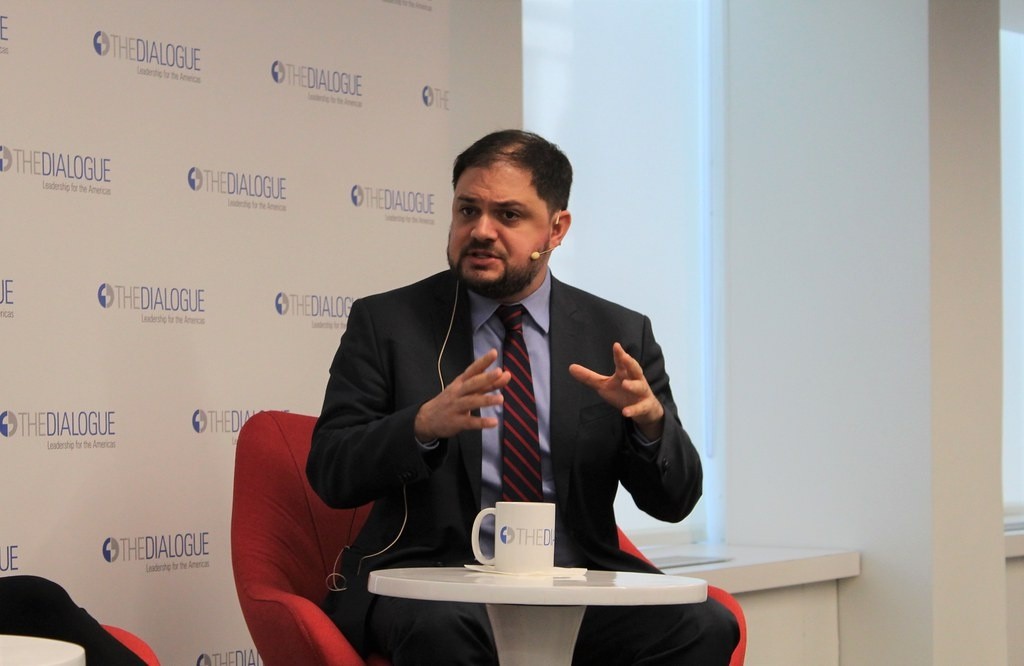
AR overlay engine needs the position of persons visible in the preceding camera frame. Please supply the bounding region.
[305,130,741,666]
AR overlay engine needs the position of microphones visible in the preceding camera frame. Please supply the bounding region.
[531,248,554,260]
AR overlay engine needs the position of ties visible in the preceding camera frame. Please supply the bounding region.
[493,304,546,502]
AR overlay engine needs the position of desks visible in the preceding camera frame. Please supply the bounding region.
[0,634,87,666]
[368,567,708,666]
[648,548,860,666]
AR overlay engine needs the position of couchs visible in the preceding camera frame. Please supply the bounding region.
[231,411,747,666]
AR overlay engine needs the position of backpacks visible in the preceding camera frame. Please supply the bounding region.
[0,575,151,666]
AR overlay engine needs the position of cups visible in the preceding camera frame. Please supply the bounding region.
[472,502,555,574]
